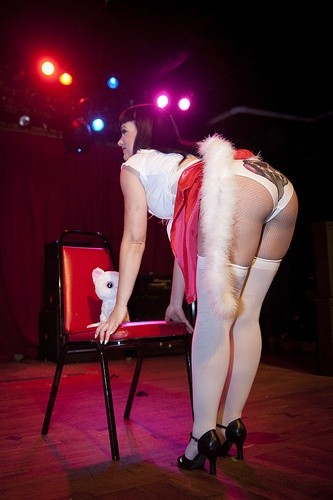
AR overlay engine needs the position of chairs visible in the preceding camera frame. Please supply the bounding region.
[43,230,194,460]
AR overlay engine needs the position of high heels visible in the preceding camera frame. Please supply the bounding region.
[216,417,248,460]
[177,429,221,475]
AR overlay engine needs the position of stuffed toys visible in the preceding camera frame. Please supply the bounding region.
[91,267,130,323]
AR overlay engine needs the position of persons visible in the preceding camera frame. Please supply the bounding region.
[87,104,298,476]
